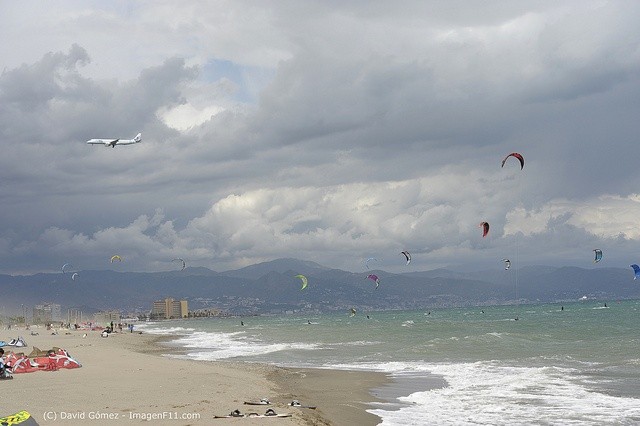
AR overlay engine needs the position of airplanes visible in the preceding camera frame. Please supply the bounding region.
[86,130,142,148]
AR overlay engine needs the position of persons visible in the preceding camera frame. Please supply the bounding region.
[111,321,135,333]
[60,322,93,330]
[0,348,14,379]
[24,322,54,328]
